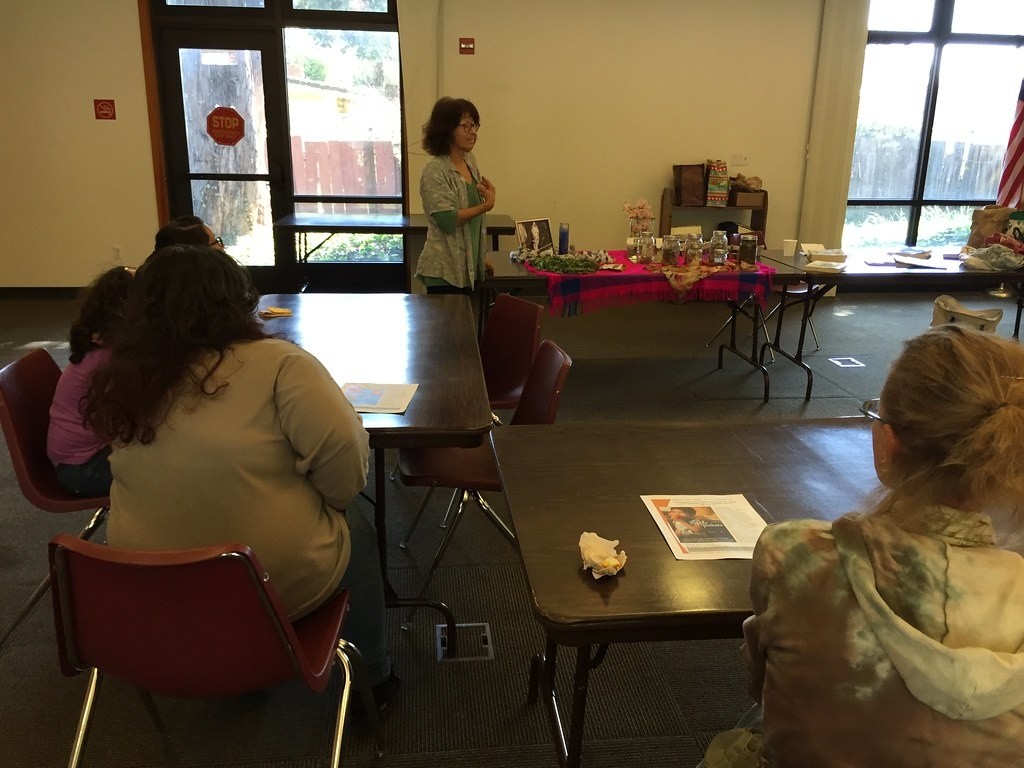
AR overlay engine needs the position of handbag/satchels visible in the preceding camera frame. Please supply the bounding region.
[929,294,1004,335]
[958,204,1024,271]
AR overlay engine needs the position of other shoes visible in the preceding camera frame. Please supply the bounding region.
[348,662,401,722]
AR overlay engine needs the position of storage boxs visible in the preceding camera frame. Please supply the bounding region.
[728,190,765,207]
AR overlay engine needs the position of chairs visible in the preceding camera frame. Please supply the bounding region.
[704,231,822,363]
[0,348,110,653]
[46,533,386,768]
[397,338,574,630]
[389,293,545,479]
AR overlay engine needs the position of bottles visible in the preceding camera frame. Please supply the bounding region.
[739,235,758,266]
[663,235,680,267]
[637,233,654,263]
[685,233,703,267]
[711,230,729,266]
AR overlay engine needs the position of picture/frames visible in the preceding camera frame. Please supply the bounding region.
[515,217,555,252]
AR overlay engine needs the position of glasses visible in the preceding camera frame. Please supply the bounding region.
[458,122,480,132]
[859,398,897,432]
[208,237,223,250]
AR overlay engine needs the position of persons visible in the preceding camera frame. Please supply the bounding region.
[669,507,709,544]
[77,242,403,731]
[417,95,495,295]
[47,265,138,499]
[697,322,1024,768]
[154,216,226,253]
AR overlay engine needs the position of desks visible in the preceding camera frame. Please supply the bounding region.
[485,251,807,404]
[659,188,769,241]
[273,213,516,293]
[252,292,494,655]
[758,247,1024,403]
[488,415,891,768]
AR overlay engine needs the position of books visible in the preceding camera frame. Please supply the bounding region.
[804,259,847,273]
[807,248,847,263]
[894,254,946,269]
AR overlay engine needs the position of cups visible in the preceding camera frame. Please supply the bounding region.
[783,240,797,257]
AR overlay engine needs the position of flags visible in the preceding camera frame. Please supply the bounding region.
[996,79,1024,209]
